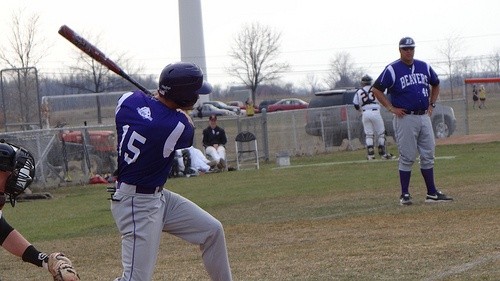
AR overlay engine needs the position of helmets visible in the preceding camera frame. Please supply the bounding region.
[158,62,203,99]
[362,75,372,84]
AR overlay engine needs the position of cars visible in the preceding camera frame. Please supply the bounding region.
[187,100,260,118]
[259,97,310,113]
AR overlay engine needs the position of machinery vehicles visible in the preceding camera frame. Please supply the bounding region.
[45,122,117,176]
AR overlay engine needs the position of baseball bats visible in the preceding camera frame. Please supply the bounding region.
[58,25,153,96]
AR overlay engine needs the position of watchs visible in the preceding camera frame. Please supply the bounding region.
[429,102,436,108]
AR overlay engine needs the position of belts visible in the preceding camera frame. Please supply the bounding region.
[403,111,424,115]
[116,181,164,195]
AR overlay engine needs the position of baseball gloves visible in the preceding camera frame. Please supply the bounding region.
[48,252,81,281]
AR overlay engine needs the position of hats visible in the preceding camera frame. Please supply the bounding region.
[209,114,217,122]
[399,37,416,48]
[0,142,16,172]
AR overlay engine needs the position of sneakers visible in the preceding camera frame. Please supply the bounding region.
[425,193,453,203]
[400,193,412,204]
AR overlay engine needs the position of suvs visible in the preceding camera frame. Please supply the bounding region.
[304,85,456,147]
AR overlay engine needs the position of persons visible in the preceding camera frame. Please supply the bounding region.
[372,37,453,205]
[0,139,82,281]
[472,85,488,110]
[353,75,393,159]
[108,62,232,281]
[203,114,226,173]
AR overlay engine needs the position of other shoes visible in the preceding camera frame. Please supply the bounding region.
[367,154,375,160]
[205,168,216,173]
[381,153,396,160]
[217,158,226,169]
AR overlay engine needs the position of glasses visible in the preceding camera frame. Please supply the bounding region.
[400,47,414,50]
